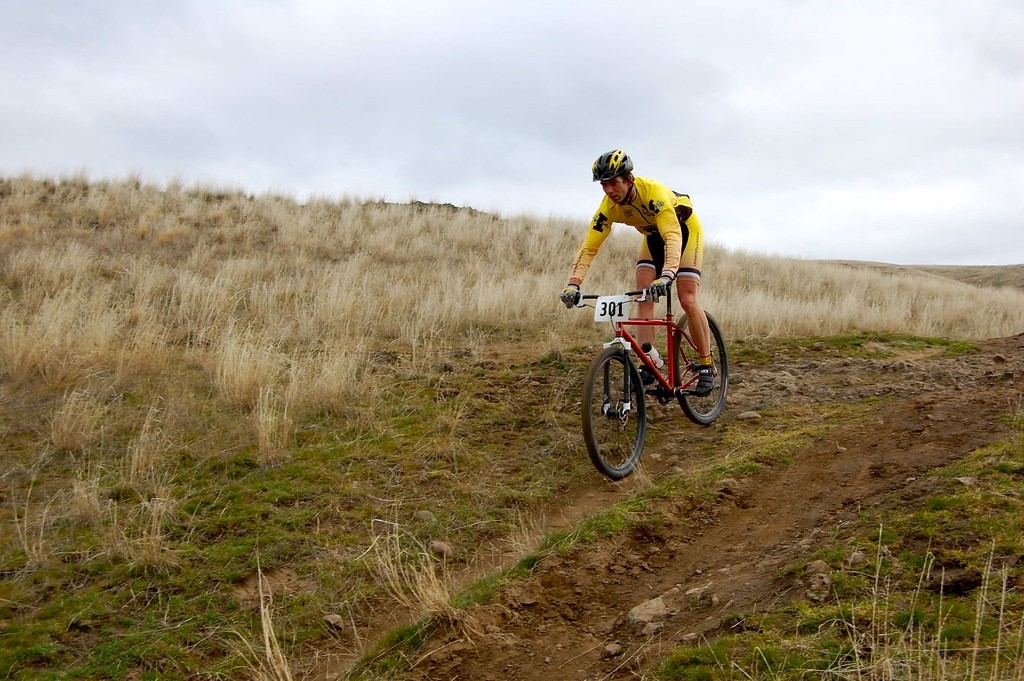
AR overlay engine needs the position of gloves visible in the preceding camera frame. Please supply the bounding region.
[561,284,581,309]
[648,277,673,303]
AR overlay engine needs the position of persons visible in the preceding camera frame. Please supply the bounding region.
[560,149,717,397]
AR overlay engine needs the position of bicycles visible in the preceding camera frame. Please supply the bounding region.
[574,278,728,481]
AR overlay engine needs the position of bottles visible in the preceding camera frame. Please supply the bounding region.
[641,342,665,370]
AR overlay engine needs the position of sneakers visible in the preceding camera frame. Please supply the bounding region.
[630,364,654,391]
[695,361,717,397]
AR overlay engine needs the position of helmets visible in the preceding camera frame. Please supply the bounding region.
[592,149,633,181]
